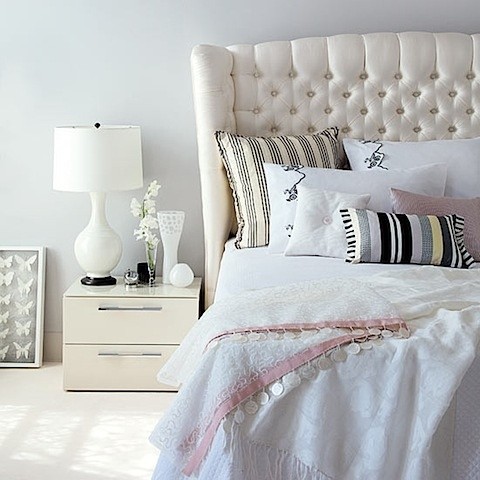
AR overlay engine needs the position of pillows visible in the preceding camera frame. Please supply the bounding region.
[213,126,480,264]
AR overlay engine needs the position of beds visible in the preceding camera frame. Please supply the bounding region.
[150,29,480,480]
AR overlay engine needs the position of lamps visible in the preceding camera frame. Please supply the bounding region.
[53,122,143,286]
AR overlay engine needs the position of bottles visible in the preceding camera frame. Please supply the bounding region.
[169,263,194,287]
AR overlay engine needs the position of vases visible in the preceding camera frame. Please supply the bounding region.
[158,207,185,284]
[145,240,158,286]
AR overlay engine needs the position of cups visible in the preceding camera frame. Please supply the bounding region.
[157,210,185,284]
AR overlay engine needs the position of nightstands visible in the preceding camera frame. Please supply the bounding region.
[63,276,205,392]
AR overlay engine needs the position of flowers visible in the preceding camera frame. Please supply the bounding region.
[131,180,161,267]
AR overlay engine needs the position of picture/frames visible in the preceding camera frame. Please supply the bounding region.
[0,244,47,368]
